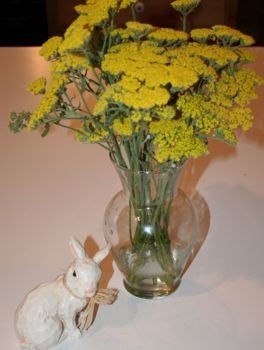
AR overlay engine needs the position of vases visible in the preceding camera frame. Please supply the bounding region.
[104,145,196,299]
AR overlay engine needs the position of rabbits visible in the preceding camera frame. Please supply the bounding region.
[13,236,111,350]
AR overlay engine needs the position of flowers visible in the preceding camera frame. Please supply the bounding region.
[8,0,264,288]
